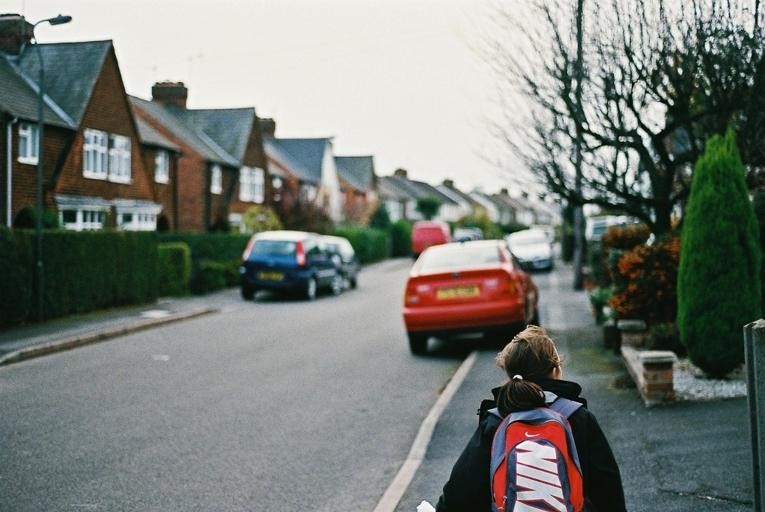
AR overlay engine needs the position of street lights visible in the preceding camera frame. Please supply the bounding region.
[31,15,72,327]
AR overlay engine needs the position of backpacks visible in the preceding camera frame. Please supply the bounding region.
[486,397,591,512]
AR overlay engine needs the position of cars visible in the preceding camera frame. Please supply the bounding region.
[240,229,361,301]
[402,222,555,355]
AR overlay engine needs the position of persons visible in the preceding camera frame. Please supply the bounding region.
[415,323,628,511]
